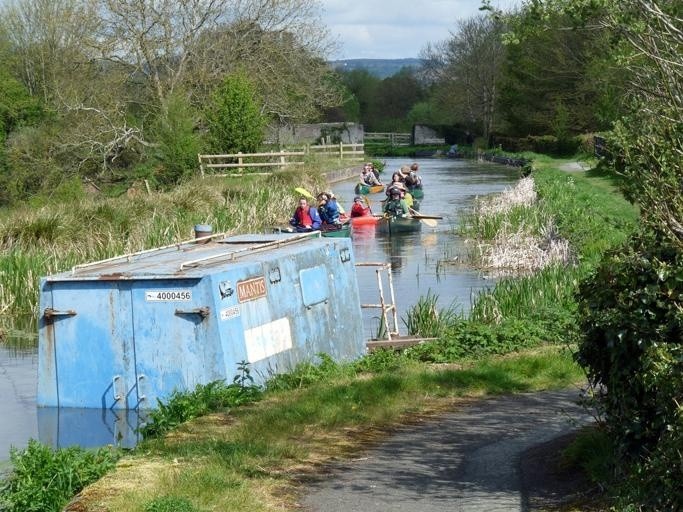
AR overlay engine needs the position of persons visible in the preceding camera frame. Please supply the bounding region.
[290,162,423,233]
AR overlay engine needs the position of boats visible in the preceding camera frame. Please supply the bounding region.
[275,182,437,237]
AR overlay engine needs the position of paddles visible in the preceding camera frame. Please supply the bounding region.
[364,197,372,214]
[295,188,317,201]
[408,206,437,227]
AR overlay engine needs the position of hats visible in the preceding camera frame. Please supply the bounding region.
[391,188,401,194]
[389,181,405,190]
